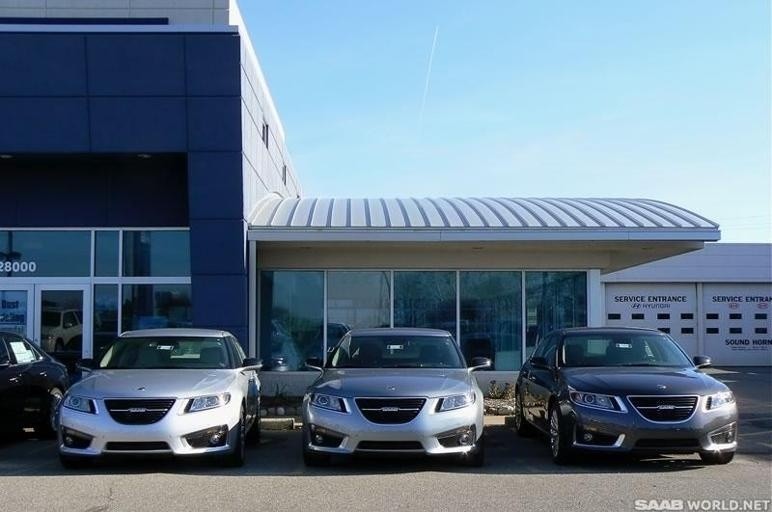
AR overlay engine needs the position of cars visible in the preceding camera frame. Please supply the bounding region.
[0,327,261,467]
[515,326,739,464]
[302,327,491,464]
[265,319,350,372]
[41,307,81,352]
